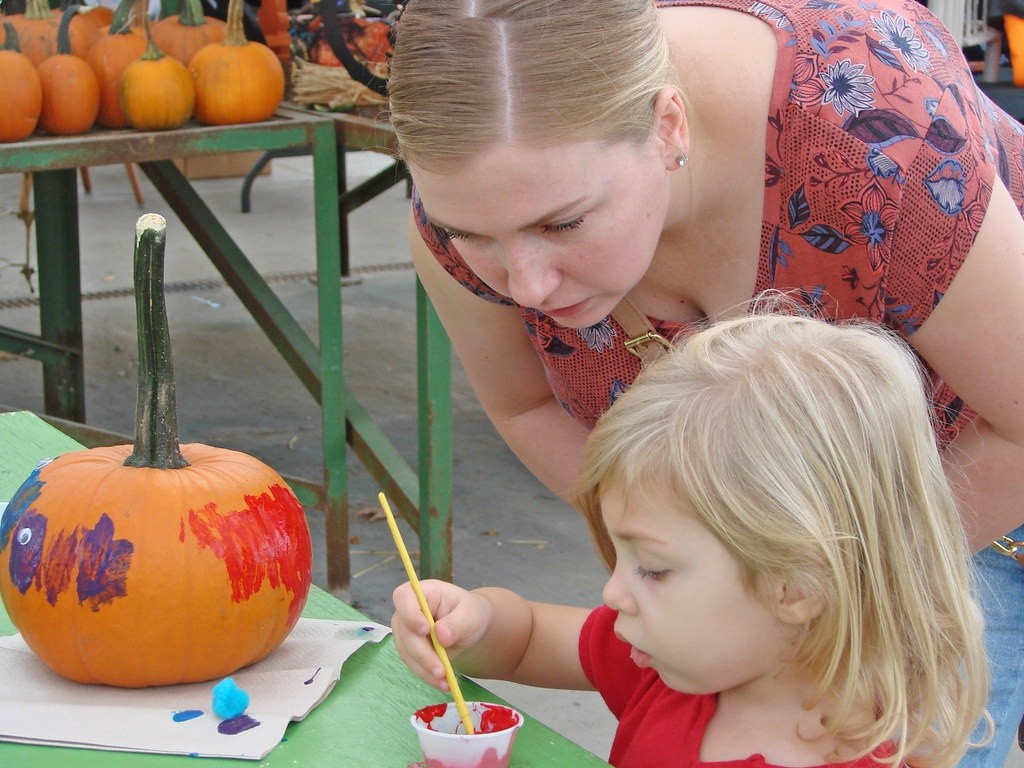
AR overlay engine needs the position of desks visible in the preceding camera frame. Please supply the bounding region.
[0,106,452,586]
[0,409,613,768]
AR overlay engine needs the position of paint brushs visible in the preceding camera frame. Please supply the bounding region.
[377,492,475,736]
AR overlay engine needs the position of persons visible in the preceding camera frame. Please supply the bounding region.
[392,314,995,768]
[387,0,1024,556]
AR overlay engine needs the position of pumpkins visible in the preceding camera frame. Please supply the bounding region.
[0,0,283,142]
[1,213,313,688]
